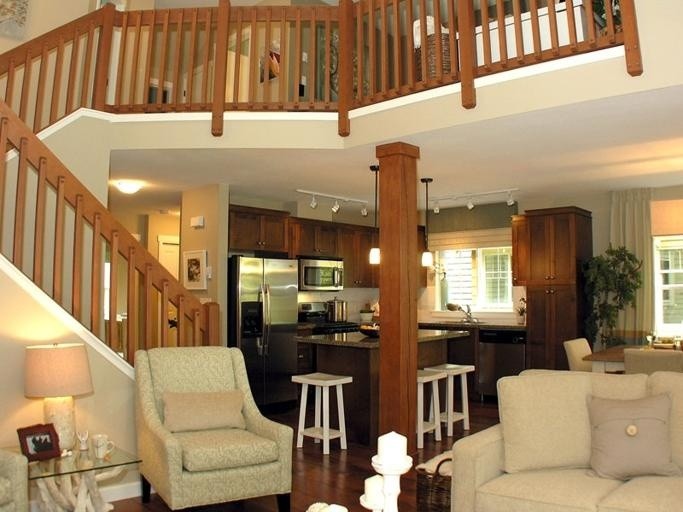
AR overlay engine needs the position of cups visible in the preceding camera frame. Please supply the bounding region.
[91,434,115,460]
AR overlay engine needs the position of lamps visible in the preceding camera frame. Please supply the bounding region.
[425,188,520,216]
[295,187,369,219]
[19,340,96,453]
[418,177,435,268]
[367,165,383,266]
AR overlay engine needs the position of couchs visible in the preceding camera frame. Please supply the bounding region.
[450,367,682,511]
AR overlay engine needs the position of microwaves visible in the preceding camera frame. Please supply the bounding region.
[299,259,345,292]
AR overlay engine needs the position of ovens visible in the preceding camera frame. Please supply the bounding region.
[476,329,525,396]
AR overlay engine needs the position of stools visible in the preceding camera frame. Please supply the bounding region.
[414,367,448,450]
[288,371,353,456]
[423,362,476,439]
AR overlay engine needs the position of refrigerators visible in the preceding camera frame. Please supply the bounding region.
[227,256,298,413]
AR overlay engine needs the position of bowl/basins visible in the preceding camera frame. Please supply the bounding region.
[359,328,379,338]
[358,312,373,322]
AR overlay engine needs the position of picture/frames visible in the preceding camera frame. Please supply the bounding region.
[180,248,208,292]
[15,422,61,463]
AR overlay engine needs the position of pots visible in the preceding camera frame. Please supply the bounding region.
[325,296,349,323]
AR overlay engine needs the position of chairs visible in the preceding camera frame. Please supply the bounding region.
[619,346,682,376]
[131,344,295,511]
[561,336,618,374]
[0,446,33,511]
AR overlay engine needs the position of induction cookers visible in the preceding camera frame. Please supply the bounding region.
[300,320,360,333]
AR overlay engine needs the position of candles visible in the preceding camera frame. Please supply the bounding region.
[360,473,387,506]
[317,502,348,512]
[373,428,409,466]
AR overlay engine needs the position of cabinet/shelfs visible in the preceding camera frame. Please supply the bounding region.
[339,221,373,289]
[371,225,428,288]
[507,206,595,285]
[522,283,593,370]
[272,216,344,263]
[227,203,291,254]
[416,322,480,403]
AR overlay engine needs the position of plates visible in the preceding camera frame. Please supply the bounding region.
[651,343,674,349]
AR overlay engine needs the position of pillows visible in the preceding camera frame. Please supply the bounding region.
[490,371,598,477]
[579,386,681,481]
[160,389,247,433]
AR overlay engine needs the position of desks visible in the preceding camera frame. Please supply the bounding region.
[291,325,471,447]
[1,431,143,512]
[452,0,609,76]
[579,342,683,376]
